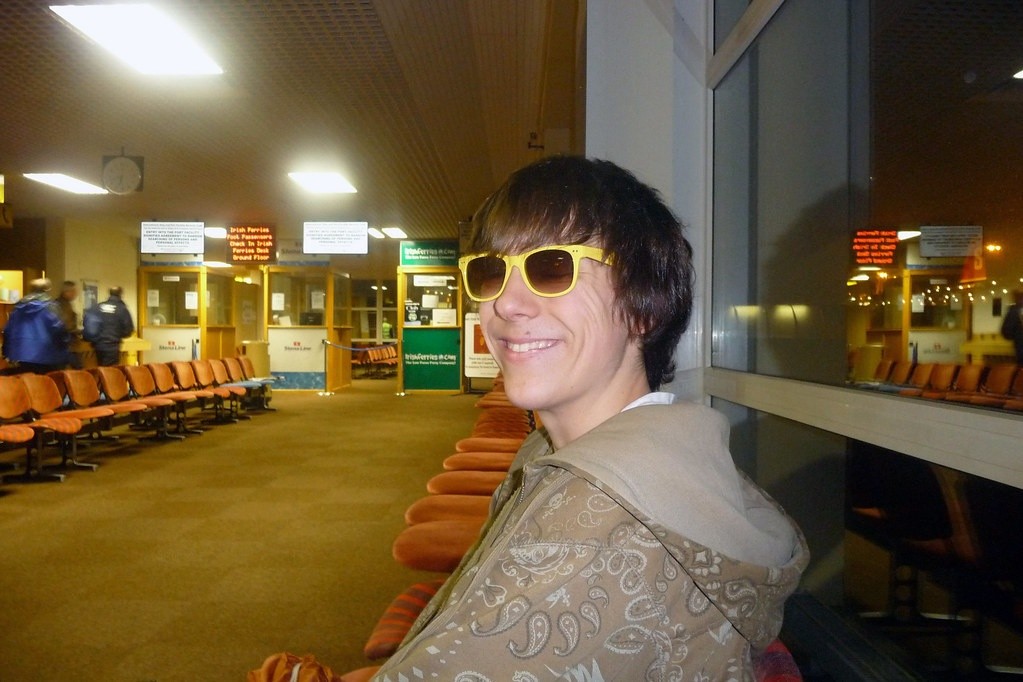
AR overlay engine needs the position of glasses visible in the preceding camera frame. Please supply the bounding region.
[459,244,625,302]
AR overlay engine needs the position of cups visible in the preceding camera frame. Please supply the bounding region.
[0,288,9,301]
[10,289,19,302]
[153,318,160,325]
[273,314,279,325]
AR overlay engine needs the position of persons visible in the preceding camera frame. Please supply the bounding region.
[54,281,84,370]
[1,277,68,374]
[371,155,810,682]
[92,287,134,367]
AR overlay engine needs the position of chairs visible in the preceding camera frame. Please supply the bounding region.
[340,342,806,682]
[852,353,1023,413]
[0,355,278,497]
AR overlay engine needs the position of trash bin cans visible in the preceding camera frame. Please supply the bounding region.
[241,340,272,410]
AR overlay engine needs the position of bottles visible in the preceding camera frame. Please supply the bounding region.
[409,306,417,322]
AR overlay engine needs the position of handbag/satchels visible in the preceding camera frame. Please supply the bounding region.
[82,308,103,341]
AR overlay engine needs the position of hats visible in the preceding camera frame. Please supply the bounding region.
[33,278,53,291]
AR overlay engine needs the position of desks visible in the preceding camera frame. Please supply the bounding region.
[69,336,152,367]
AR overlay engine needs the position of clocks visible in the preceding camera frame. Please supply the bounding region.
[103,145,145,195]
